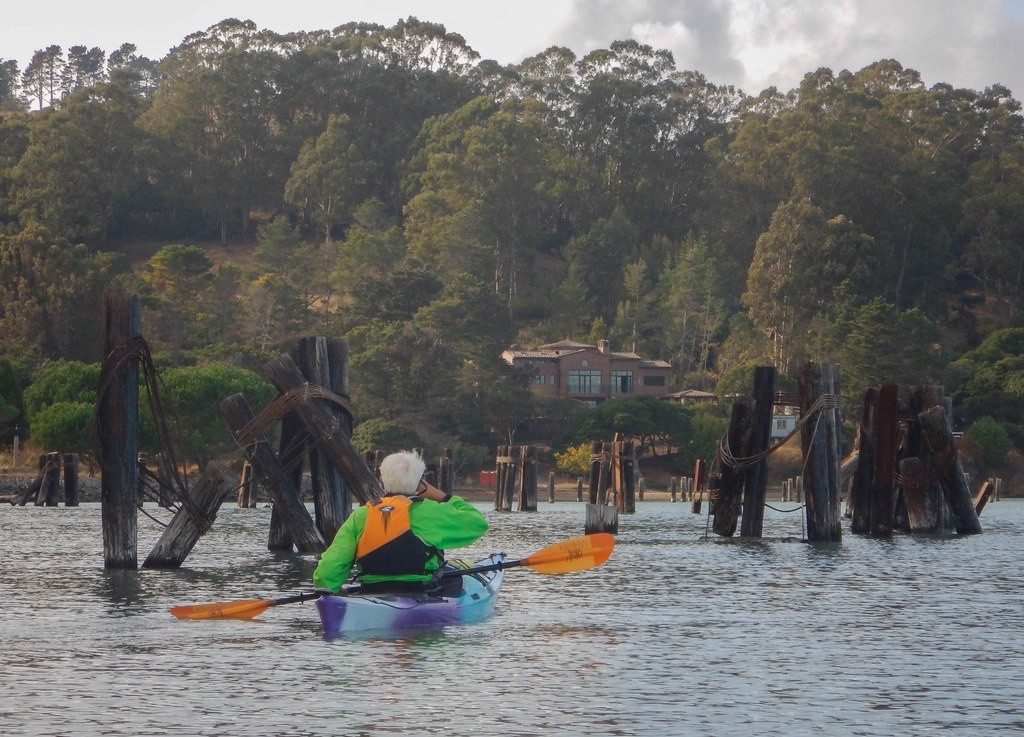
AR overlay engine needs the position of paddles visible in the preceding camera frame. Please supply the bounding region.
[169,531,617,621]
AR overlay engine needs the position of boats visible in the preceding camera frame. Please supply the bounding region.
[315,552,509,639]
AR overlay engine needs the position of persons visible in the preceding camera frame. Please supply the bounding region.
[312,449,489,596]
